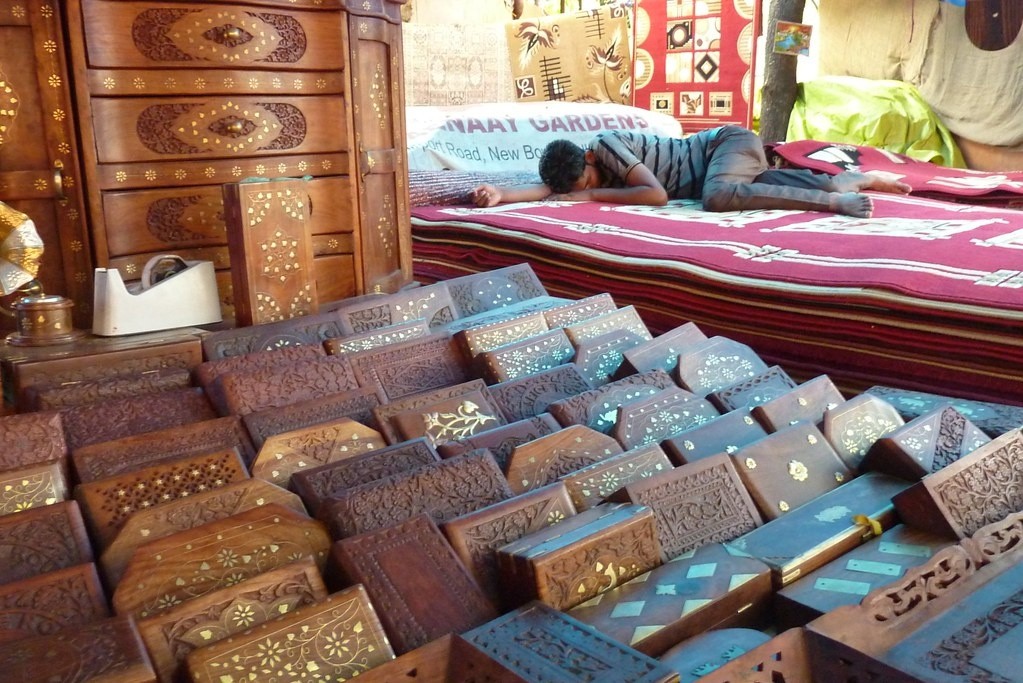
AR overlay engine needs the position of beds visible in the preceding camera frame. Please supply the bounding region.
[397,102,1023,410]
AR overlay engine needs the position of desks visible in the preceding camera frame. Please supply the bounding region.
[0,328,214,414]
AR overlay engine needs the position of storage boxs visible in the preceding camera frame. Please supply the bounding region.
[0,262,1023,683]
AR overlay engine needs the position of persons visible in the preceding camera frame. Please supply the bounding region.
[473,125,913,219]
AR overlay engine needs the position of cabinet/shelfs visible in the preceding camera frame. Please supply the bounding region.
[65,0,420,320]
[0,0,95,327]
